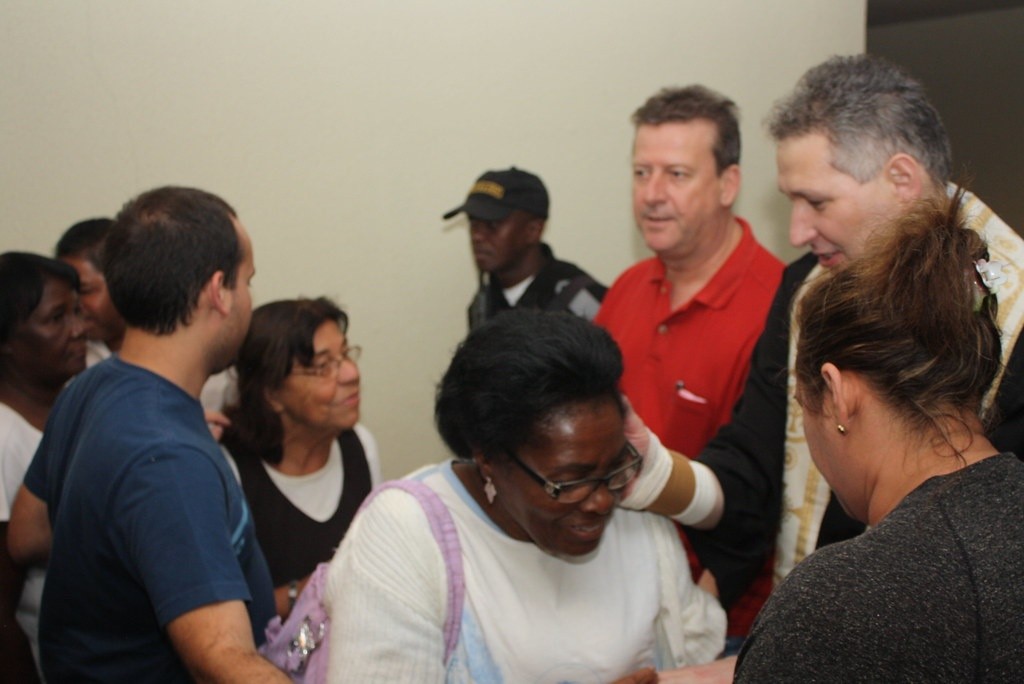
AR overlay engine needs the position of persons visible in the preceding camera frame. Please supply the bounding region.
[0,251,88,684]
[728,195,1024,684]
[322,307,692,684]
[217,297,382,624]
[8,187,292,684]
[619,56,1024,684]
[589,86,786,659]
[442,164,607,331]
[56,217,127,368]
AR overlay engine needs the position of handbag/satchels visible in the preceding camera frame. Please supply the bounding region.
[256,478,466,684]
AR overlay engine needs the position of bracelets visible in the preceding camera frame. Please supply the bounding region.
[288,580,297,609]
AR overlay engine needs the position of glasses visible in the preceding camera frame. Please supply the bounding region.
[290,345,362,376]
[508,441,644,506]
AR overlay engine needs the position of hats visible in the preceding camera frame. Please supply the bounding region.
[443,167,550,220]
